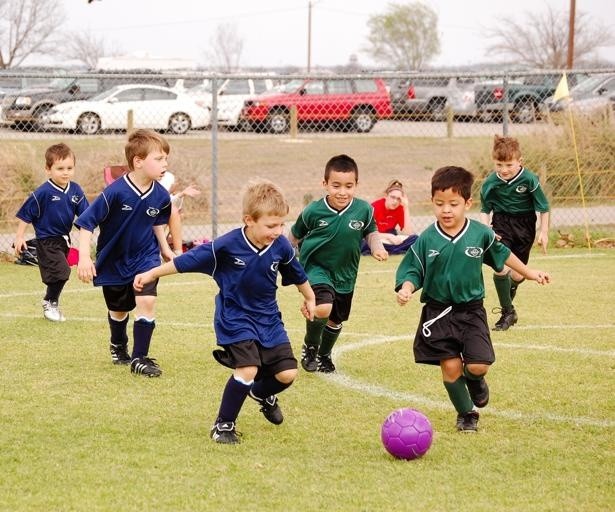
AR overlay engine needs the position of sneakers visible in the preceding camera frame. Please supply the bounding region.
[109,341,132,366]
[510,283,518,300]
[41,299,60,322]
[456,411,478,434]
[467,379,490,408]
[301,339,321,372]
[492,306,519,332]
[130,355,163,378]
[317,352,335,373]
[210,417,240,444]
[248,389,284,424]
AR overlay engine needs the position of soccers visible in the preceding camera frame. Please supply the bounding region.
[382,410,431,459]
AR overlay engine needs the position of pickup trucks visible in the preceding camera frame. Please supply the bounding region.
[473,73,594,123]
[193,73,325,127]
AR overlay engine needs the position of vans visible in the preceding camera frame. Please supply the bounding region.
[391,76,523,121]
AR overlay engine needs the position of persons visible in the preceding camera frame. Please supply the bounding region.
[360,179,418,257]
[14,144,89,322]
[153,173,201,260]
[133,180,315,447]
[395,166,549,433]
[290,154,389,373]
[480,135,549,331]
[74,129,178,376]
[400,80,416,99]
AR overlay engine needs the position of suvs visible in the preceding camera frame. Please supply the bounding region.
[0,69,171,131]
[239,78,394,134]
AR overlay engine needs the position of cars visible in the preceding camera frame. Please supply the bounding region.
[544,72,615,113]
[173,72,208,93]
[40,83,211,136]
